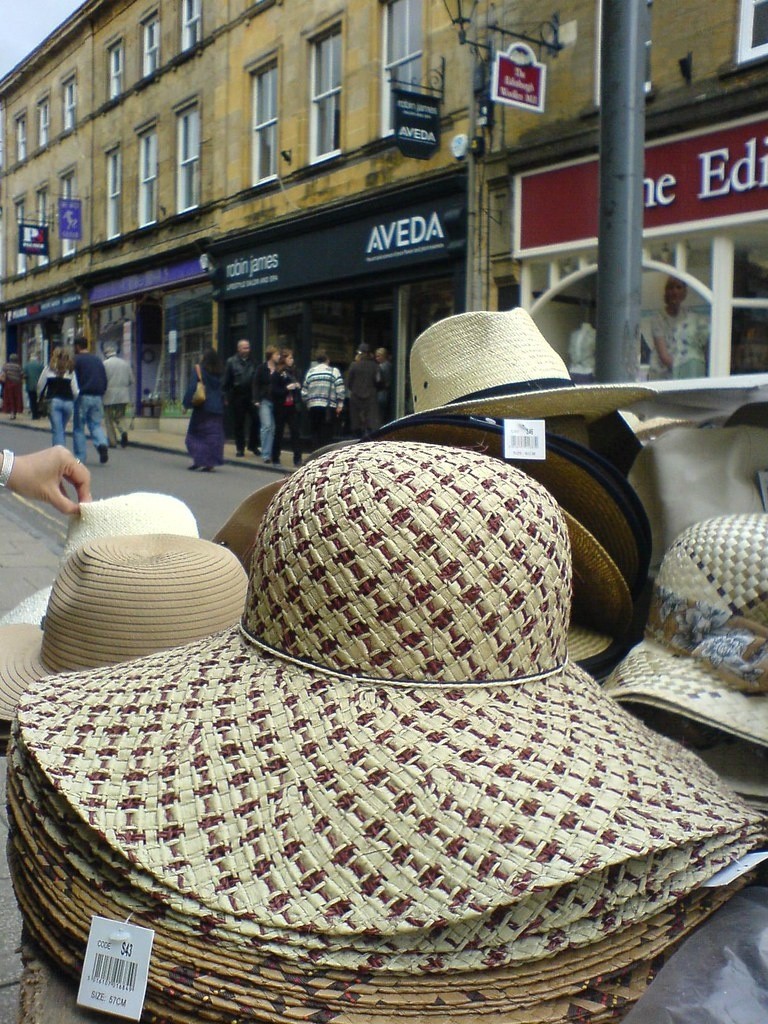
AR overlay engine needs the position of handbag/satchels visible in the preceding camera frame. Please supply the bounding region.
[37,382,49,418]
[190,365,207,405]
[325,404,340,425]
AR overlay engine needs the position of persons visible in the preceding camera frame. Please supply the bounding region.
[0,445,92,514]
[0,354,23,418]
[35,346,80,448]
[268,350,304,466]
[73,337,108,464]
[183,348,224,470]
[569,323,595,383]
[649,278,708,379]
[300,354,345,450]
[345,343,393,437]
[252,345,281,464]
[102,341,134,448]
[23,353,44,420]
[222,339,263,457]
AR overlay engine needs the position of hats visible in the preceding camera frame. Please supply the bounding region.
[0,307,768,1024]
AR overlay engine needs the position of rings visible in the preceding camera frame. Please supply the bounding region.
[77,459,80,464]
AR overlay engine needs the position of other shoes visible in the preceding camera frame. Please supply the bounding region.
[235,449,244,457]
[108,442,117,448]
[292,462,303,467]
[121,431,127,449]
[200,468,207,471]
[251,448,261,456]
[262,456,271,464]
[186,464,198,471]
[272,461,281,467]
[98,445,108,464]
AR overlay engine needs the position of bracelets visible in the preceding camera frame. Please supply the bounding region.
[0,449,14,488]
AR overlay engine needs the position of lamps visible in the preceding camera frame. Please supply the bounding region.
[443,0,493,62]
